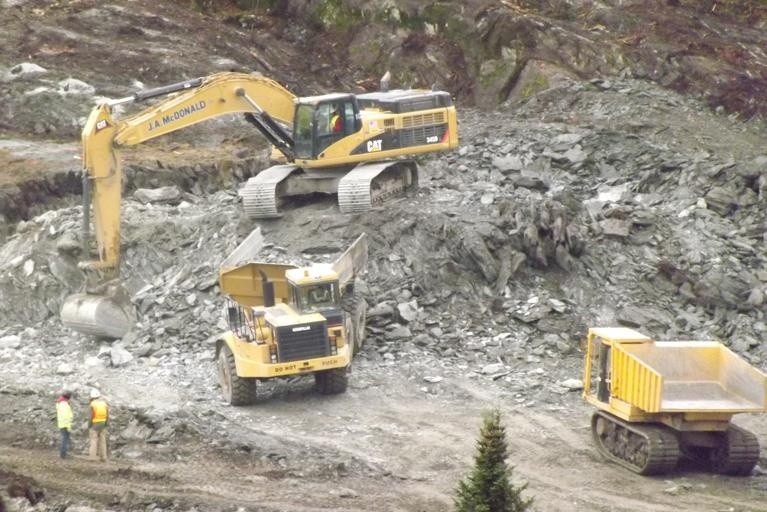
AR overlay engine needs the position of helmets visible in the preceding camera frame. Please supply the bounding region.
[89,388,100,399]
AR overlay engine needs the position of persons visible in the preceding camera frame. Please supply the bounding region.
[55,391,74,459]
[86,388,110,461]
[326,107,342,134]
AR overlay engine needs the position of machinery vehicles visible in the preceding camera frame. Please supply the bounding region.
[578,324,767,475]
[206,225,371,407]
[59,69,459,343]
